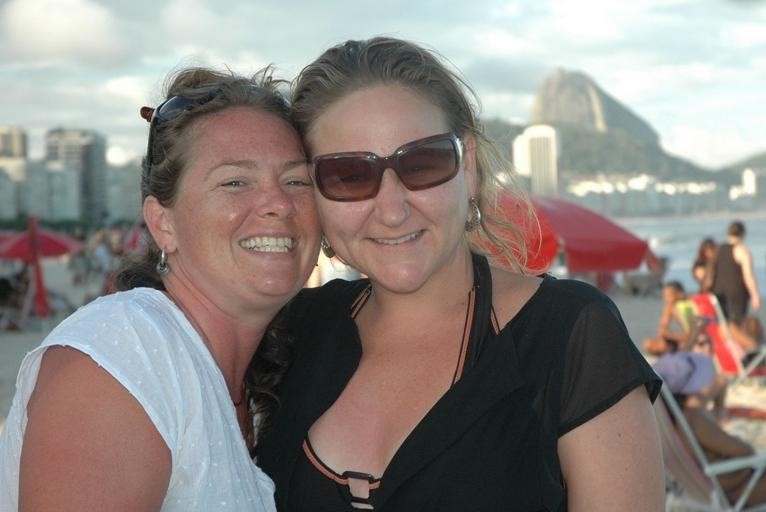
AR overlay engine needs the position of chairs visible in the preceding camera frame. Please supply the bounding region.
[694,293,765,385]
[648,370,764,512]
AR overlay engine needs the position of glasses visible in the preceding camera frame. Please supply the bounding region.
[149,83,290,156]
[310,132,464,202]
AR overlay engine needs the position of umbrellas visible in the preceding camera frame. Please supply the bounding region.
[474,181,645,280]
[1,227,84,280]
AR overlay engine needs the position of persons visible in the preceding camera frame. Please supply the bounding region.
[230,35,671,510]
[641,221,766,507]
[1,68,325,510]
